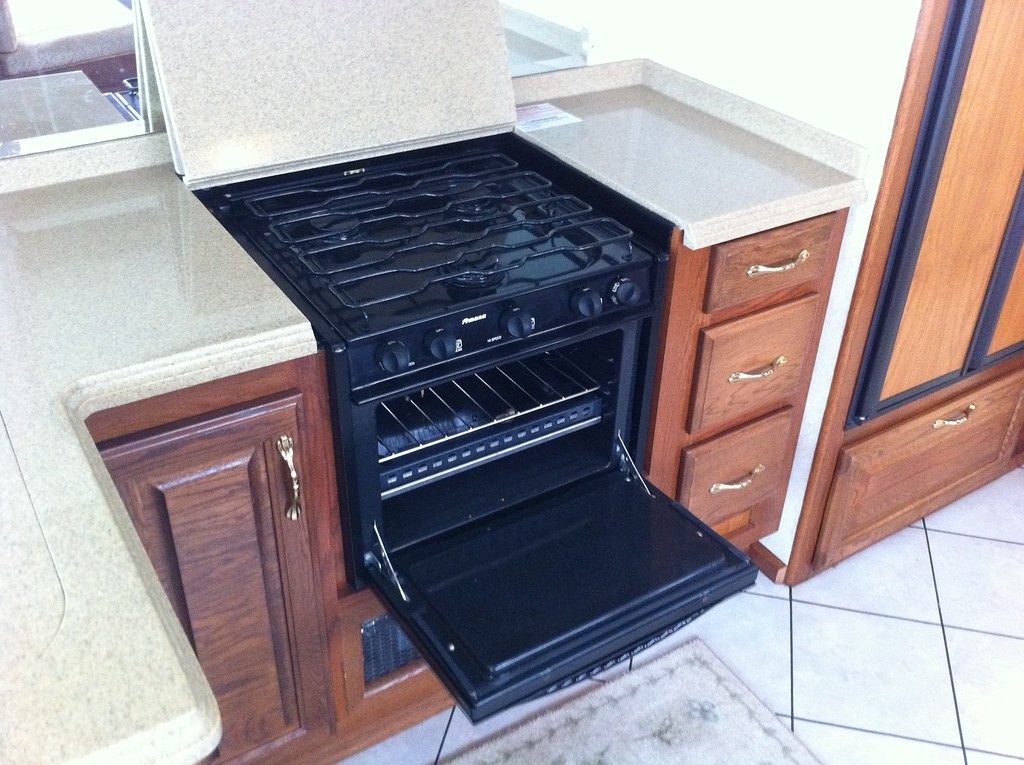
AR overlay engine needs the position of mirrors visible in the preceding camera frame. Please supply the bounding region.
[0,0,646,161]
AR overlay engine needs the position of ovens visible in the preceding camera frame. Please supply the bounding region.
[332,315,766,727]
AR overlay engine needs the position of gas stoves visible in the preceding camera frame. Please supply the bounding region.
[212,149,668,407]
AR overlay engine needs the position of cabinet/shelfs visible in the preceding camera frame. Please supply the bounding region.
[642,206,851,551]
[786,0,1024,589]
[84,349,349,765]
